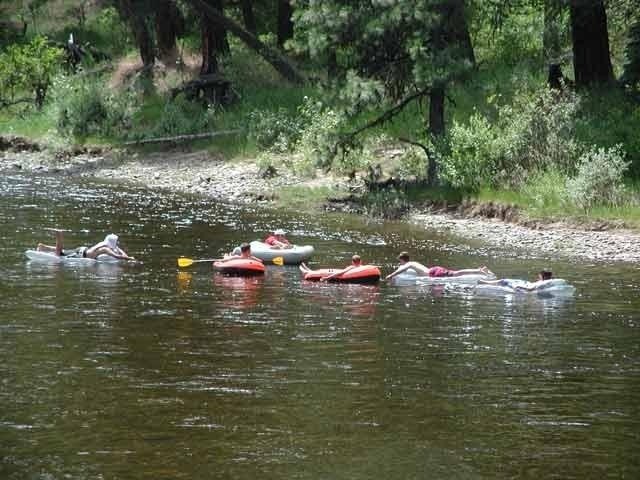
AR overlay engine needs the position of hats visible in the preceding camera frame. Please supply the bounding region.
[274,229,288,236]
[396,250,411,259]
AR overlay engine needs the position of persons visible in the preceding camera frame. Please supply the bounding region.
[299,255,383,282]
[477,268,553,292]
[37,230,135,261]
[223,243,262,262]
[385,252,495,280]
[265,229,293,249]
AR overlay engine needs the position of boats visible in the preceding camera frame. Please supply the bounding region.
[471,283,579,298]
[306,265,383,283]
[246,240,316,262]
[213,258,266,274]
[27,248,136,270]
[393,267,497,284]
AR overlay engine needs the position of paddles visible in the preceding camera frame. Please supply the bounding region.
[178,257,284,267]
